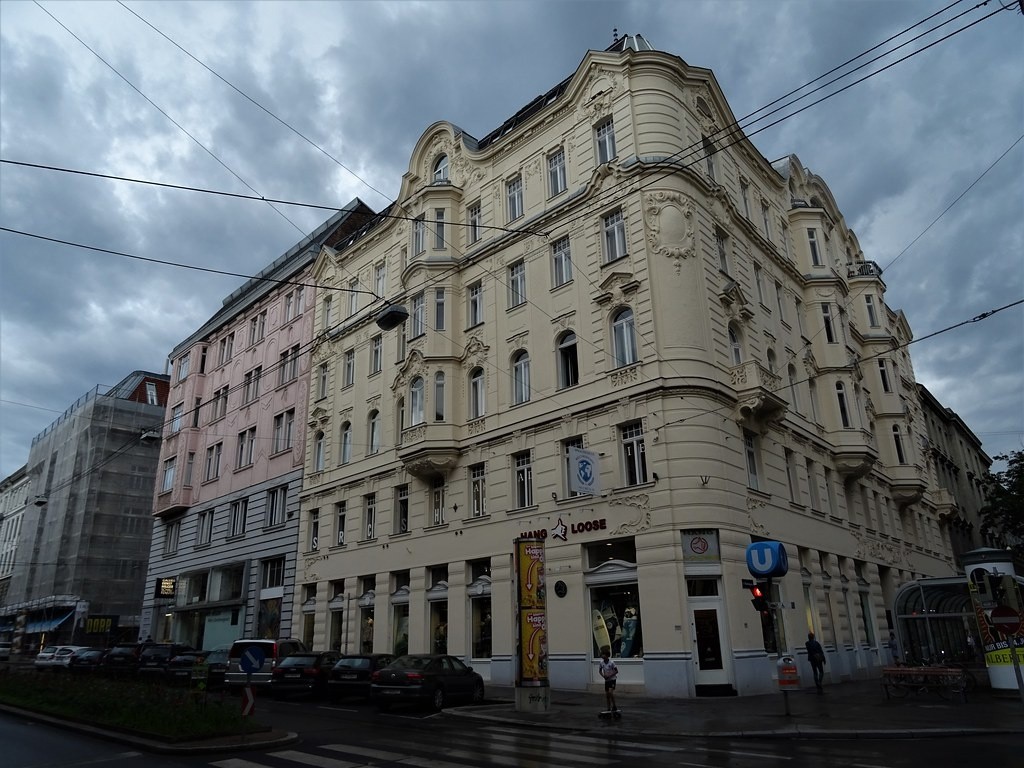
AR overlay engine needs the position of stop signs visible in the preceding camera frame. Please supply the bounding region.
[991,605,1021,636]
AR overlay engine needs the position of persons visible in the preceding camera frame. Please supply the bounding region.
[888,631,899,661]
[805,633,826,690]
[137,637,144,644]
[968,636,975,659]
[599,651,618,711]
[145,635,153,642]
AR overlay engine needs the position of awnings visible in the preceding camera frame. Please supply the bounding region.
[0,610,74,632]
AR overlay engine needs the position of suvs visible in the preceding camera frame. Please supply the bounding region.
[228,636,308,687]
[106,641,158,676]
[138,643,196,680]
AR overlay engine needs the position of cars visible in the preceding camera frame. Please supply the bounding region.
[0,642,12,660]
[169,649,233,686]
[271,650,350,698]
[372,652,485,709]
[35,644,110,674]
[329,652,409,696]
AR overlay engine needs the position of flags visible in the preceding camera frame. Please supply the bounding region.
[569,446,602,497]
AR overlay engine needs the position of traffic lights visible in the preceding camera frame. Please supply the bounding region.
[747,581,767,612]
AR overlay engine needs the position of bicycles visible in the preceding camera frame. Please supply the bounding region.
[885,649,971,704]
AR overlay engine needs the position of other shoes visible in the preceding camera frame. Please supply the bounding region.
[600,710,612,714]
[611,709,622,713]
